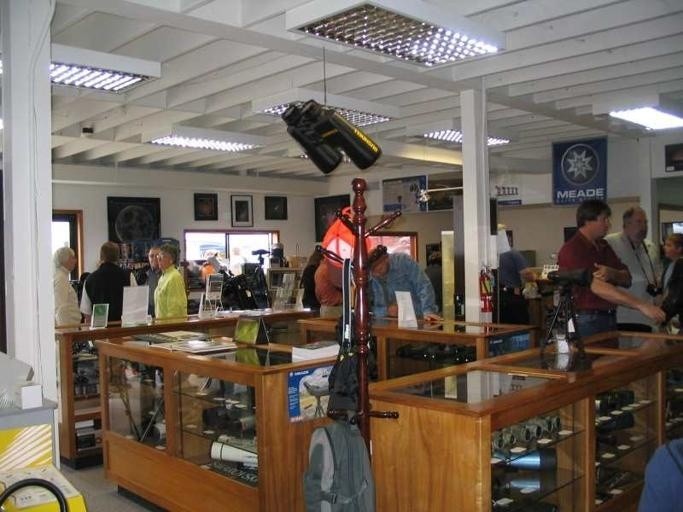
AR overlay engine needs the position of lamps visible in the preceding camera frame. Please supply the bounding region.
[418,186,463,203]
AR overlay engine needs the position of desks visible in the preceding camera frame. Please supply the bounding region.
[0,394,59,474]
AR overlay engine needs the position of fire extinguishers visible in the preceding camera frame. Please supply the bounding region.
[479,262,495,312]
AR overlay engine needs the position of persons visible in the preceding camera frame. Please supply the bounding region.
[53,238,188,326]
[492,249,537,324]
[300,245,443,321]
[659,234,683,329]
[603,206,664,333]
[637,438,683,511]
[207,252,229,277]
[557,200,666,348]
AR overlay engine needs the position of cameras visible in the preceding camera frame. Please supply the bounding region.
[548,270,593,287]
[645,283,663,297]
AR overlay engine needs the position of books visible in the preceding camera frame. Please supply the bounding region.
[290,340,340,359]
[134,330,240,354]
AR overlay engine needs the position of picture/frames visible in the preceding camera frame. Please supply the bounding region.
[231,194,254,227]
[315,194,350,242]
[52,209,84,286]
[364,231,418,265]
[265,196,288,220]
[194,193,217,220]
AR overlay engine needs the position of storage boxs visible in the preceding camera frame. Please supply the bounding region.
[0,463,87,512]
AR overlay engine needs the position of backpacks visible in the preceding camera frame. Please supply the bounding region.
[302,418,377,512]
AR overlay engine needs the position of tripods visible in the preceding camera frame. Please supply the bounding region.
[543,290,587,357]
[248,256,272,308]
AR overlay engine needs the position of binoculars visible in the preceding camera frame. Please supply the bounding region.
[491,415,561,450]
[281,99,382,173]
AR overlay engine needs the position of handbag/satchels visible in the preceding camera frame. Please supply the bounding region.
[328,337,359,419]
